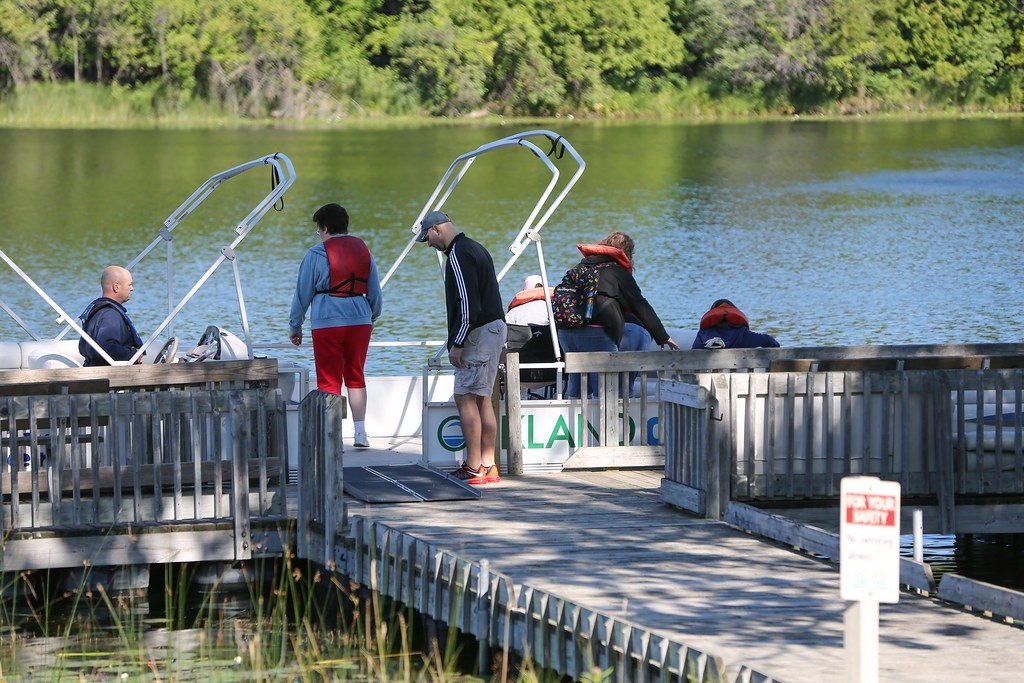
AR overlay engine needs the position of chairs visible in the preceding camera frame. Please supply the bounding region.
[498,322,570,400]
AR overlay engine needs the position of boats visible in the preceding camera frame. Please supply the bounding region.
[0,129,1024,481]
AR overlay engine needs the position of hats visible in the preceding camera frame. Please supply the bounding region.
[415,211,452,242]
[523,274,544,290]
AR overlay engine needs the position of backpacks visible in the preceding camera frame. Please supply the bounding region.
[551,262,618,326]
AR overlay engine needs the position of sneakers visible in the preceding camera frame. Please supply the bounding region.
[450,460,500,484]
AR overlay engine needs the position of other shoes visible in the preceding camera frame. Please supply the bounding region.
[342,442,345,454]
[354,433,369,447]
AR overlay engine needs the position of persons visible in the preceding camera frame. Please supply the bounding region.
[691,299,779,349]
[417,212,508,484]
[79,266,145,367]
[557,231,680,399]
[288,203,383,447]
[505,276,557,326]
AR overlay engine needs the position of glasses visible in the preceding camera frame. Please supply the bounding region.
[316,229,321,236]
